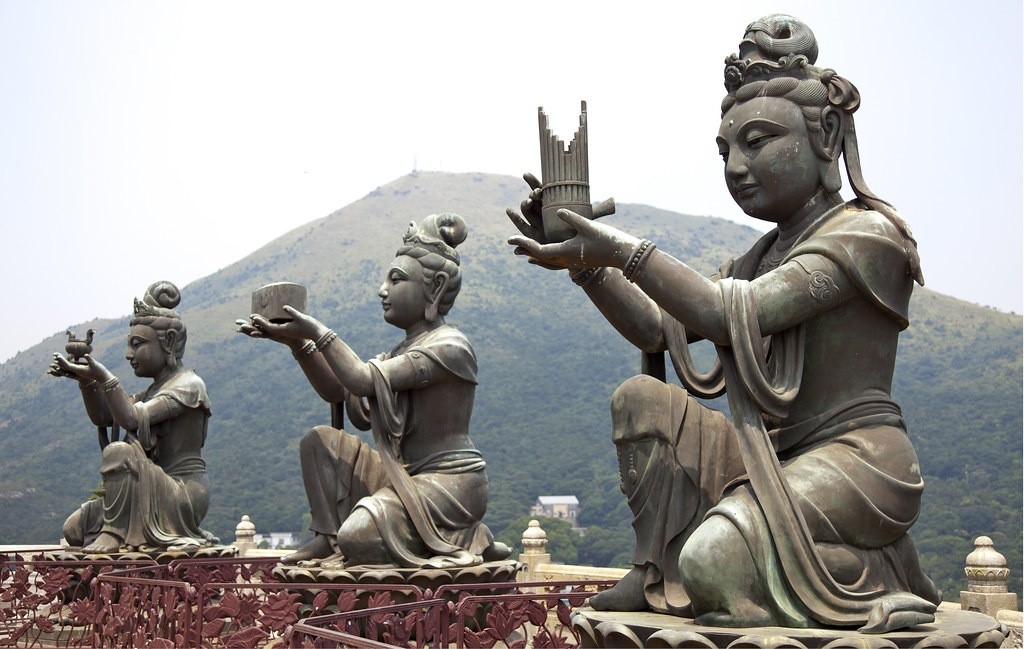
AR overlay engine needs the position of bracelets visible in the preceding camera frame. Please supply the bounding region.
[79,380,99,393]
[292,340,316,361]
[572,265,603,287]
[622,239,656,284]
[100,376,119,392]
[315,329,337,351]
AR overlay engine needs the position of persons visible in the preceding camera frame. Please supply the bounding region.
[507,15,943,629]
[47,279,220,553]
[236,212,513,570]
